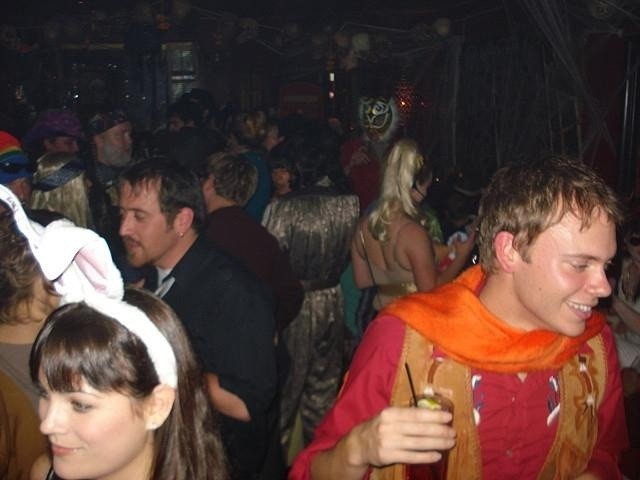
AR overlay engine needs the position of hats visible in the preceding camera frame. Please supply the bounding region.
[76,94,128,139]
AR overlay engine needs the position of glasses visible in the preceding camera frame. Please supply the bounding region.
[0,159,36,175]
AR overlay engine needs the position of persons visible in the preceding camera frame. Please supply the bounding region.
[29,284,233,480]
[1,206,77,480]
[117,157,284,480]
[283,149,633,480]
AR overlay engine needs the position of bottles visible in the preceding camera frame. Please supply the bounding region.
[437,253,455,272]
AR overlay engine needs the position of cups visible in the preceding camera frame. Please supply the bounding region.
[404,394,455,480]
